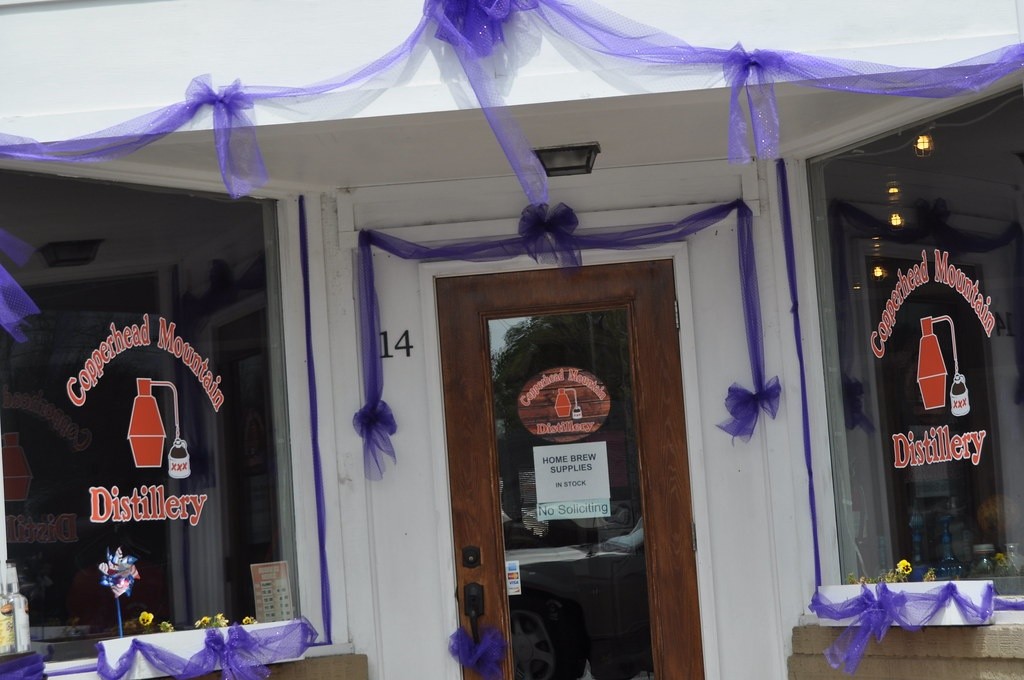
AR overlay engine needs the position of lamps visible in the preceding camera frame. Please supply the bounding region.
[533,141,601,178]
[868,262,889,282]
[913,132,936,158]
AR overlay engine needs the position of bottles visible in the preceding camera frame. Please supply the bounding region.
[6,560,31,654]
[907,511,932,582]
[995,543,1024,595]
[935,517,964,581]
[0,560,18,656]
[969,544,996,580]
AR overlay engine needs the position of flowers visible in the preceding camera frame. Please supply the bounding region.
[844,559,913,584]
[160,621,175,633]
[194,613,229,629]
[137,610,154,635]
[243,617,258,625]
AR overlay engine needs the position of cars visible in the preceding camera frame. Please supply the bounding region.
[20,524,173,635]
[506,502,655,680]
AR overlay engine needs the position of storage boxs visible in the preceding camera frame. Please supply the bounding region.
[818,580,996,626]
[97,620,305,680]
[991,595,1024,624]
